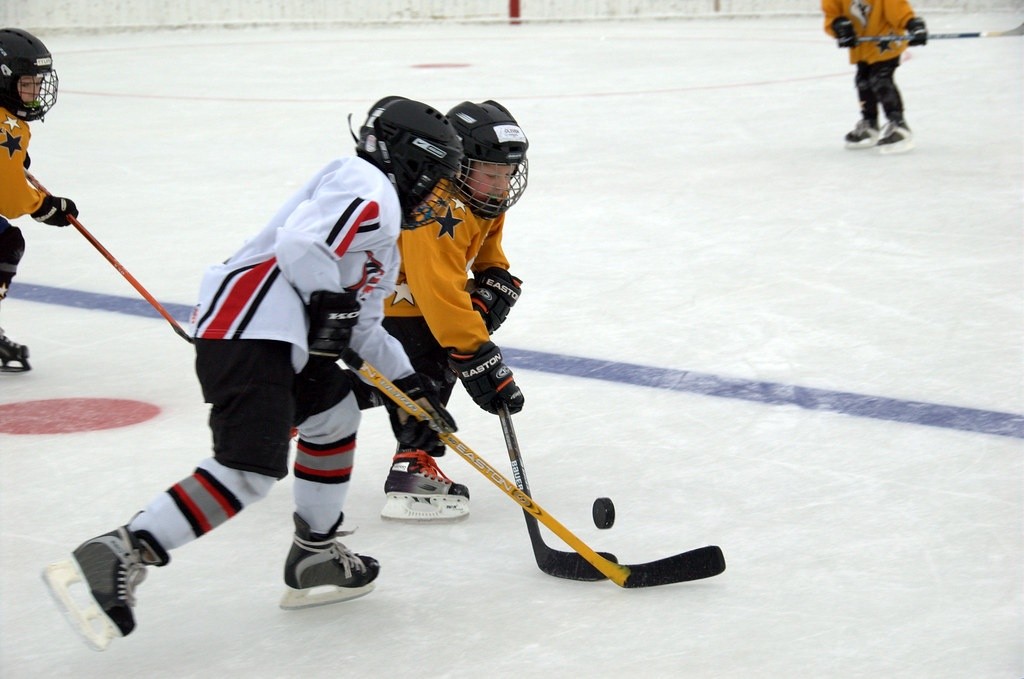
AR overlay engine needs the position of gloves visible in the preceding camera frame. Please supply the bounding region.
[470,266,523,335]
[30,196,78,227]
[306,291,361,380]
[832,16,856,47]
[449,341,524,414]
[905,16,926,45]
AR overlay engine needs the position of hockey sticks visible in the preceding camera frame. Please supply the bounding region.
[342,347,726,587]
[856,21,1024,39]
[26,167,195,345]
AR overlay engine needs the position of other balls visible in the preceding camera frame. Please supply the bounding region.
[594,498,615,530]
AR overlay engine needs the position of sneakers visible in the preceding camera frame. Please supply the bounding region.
[280,512,381,608]
[844,111,879,148]
[42,509,171,653]
[876,112,913,152]
[382,448,471,520]
[0,328,31,372]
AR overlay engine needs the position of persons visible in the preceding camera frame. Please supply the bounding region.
[821,0,928,146]
[0,27,80,360]
[72,99,529,637]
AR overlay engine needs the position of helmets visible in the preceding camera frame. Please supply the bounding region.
[347,95,466,231]
[445,97,529,216]
[0,28,58,126]
